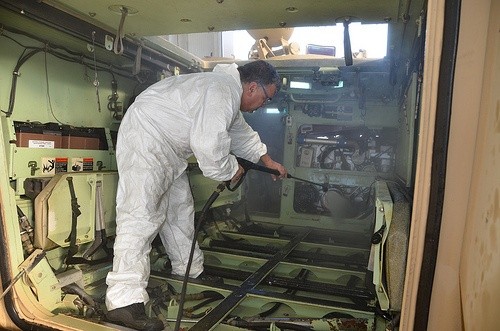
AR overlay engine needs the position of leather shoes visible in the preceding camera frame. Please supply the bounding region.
[104,302,165,330]
[172,270,224,288]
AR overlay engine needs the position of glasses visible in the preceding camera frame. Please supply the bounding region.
[259,82,271,106]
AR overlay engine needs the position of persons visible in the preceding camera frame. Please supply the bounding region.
[105,59,288,331]
